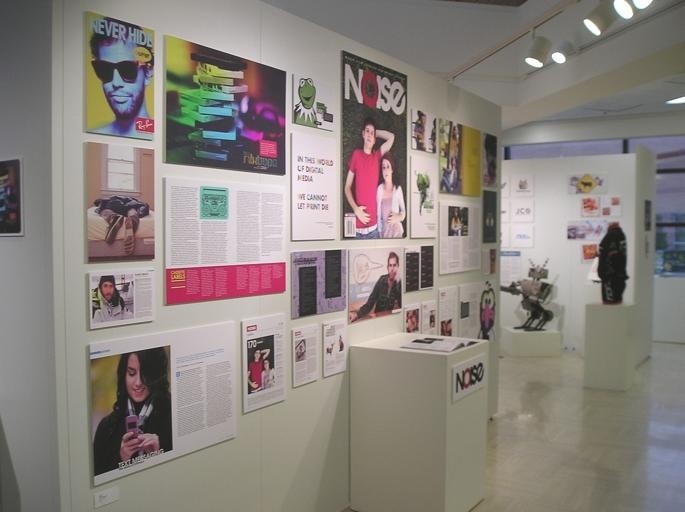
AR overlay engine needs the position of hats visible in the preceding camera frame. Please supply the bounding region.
[98,276,116,288]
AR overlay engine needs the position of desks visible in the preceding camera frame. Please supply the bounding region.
[352,332,488,511]
[654,275,685,343]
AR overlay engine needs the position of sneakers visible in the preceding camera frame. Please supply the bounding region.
[105,215,123,242]
[125,217,135,256]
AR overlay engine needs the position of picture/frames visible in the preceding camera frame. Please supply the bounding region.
[0,156,24,237]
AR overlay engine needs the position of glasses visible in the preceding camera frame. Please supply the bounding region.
[91,60,138,84]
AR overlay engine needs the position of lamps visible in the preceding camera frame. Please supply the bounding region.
[632,0,655,11]
[613,0,638,20]
[525,25,551,68]
[555,42,574,64]
[583,0,618,36]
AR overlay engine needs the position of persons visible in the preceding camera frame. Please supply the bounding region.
[245,347,276,394]
[92,194,149,250]
[451,207,469,236]
[441,156,459,192]
[87,16,154,139]
[599,224,630,304]
[345,119,408,239]
[350,252,402,320]
[414,110,428,150]
[91,275,132,320]
[94,348,174,475]
